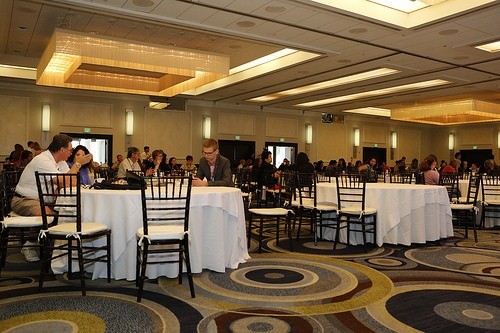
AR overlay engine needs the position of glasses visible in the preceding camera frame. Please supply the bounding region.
[65,148,73,153]
[202,149,216,156]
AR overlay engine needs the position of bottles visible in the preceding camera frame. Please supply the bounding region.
[463,173,465,180]
[468,172,471,180]
[466,174,468,180]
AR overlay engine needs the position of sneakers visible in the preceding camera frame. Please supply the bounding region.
[20,244,40,263]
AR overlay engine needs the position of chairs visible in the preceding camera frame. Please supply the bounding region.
[34,171,111,296]
[247,168,500,252]
[0,173,54,270]
[135,173,195,303]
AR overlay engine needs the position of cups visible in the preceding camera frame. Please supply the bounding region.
[184,179,190,185]
[97,178,105,183]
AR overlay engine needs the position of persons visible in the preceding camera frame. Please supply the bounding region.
[395,154,440,185]
[11,134,93,261]
[192,140,235,186]
[438,152,500,176]
[313,157,381,183]
[9,141,44,182]
[66,145,91,184]
[238,151,285,189]
[279,152,314,192]
[112,146,198,178]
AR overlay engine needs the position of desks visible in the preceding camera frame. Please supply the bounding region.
[315,182,454,247]
[453,180,499,228]
[55,184,251,280]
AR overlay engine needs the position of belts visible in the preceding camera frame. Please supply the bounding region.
[13,192,24,198]
